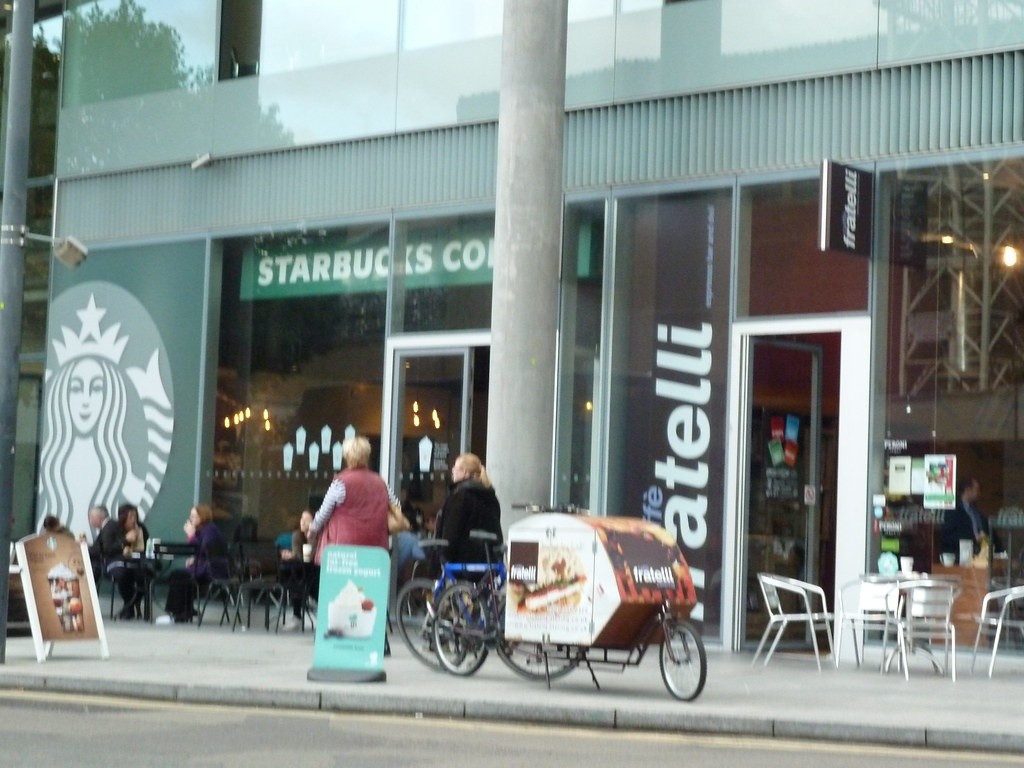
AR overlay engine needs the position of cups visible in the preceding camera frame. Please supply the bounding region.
[943,553,955,566]
[303,544,312,562]
[901,557,913,575]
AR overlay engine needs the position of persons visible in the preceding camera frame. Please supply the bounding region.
[306,437,404,658]
[81,502,150,618]
[156,503,228,624]
[938,472,1003,565]
[43,514,74,540]
[275,508,314,632]
[436,451,503,585]
[393,508,437,569]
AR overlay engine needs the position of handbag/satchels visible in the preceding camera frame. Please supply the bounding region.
[386,506,411,533]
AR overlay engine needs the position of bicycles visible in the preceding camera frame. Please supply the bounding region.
[394,502,707,703]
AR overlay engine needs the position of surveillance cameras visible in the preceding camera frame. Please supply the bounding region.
[191,153,212,171]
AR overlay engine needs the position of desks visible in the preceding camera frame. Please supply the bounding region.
[929,560,990,647]
[864,573,960,674]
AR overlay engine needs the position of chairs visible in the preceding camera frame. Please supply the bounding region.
[753,573,836,672]
[97,541,392,653]
[836,577,1024,683]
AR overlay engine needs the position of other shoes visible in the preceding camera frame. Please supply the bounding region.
[281,615,301,632]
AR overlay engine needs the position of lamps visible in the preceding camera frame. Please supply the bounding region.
[190,152,212,171]
